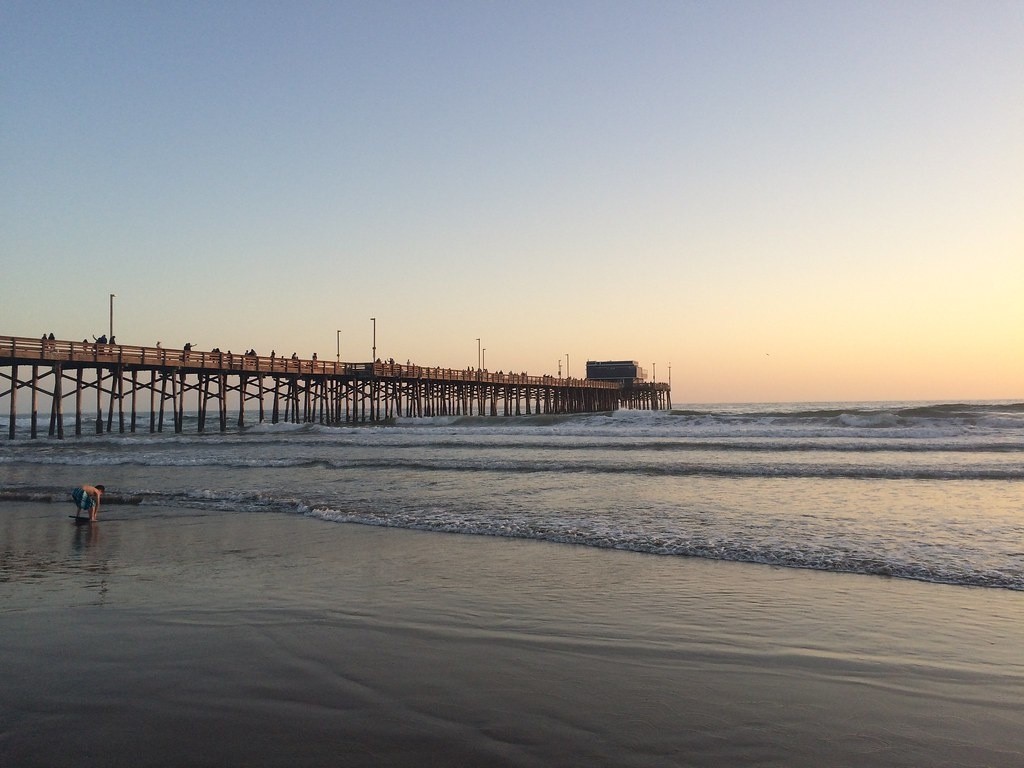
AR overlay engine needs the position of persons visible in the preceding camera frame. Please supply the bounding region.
[72,484,105,522]
[376,357,556,385]
[91,334,116,354]
[48,333,55,349]
[156,341,318,370]
[42,334,47,340]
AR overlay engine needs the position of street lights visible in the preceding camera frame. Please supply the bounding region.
[337,330,341,362]
[110,294,115,337]
[370,318,376,362]
[476,338,480,371]
[483,349,485,371]
